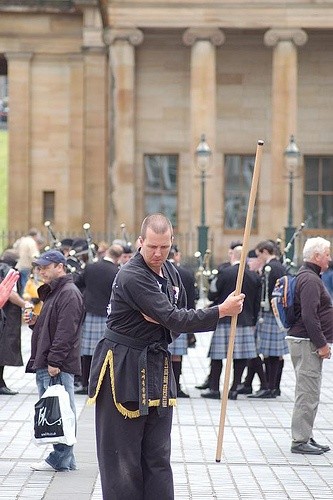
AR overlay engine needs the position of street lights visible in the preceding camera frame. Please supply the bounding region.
[282,134,302,263]
[195,134,212,269]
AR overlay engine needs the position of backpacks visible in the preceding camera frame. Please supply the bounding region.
[271,269,322,333]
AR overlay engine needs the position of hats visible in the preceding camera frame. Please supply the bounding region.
[31,250,67,266]
[123,246,133,253]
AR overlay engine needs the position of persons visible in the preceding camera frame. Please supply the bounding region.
[0,228,333,401]
[24,250,84,472]
[286,237,333,455]
[87,214,245,500]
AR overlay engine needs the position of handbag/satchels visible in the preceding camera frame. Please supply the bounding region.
[34,373,79,447]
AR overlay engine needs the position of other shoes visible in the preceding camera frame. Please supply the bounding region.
[0,386,18,395]
[247,388,271,399]
[228,391,237,399]
[271,389,276,398]
[74,385,88,394]
[194,381,210,390]
[178,390,190,397]
[237,384,252,394]
[30,461,56,471]
[276,388,280,395]
[307,438,331,452]
[291,442,323,455]
[201,391,221,399]
